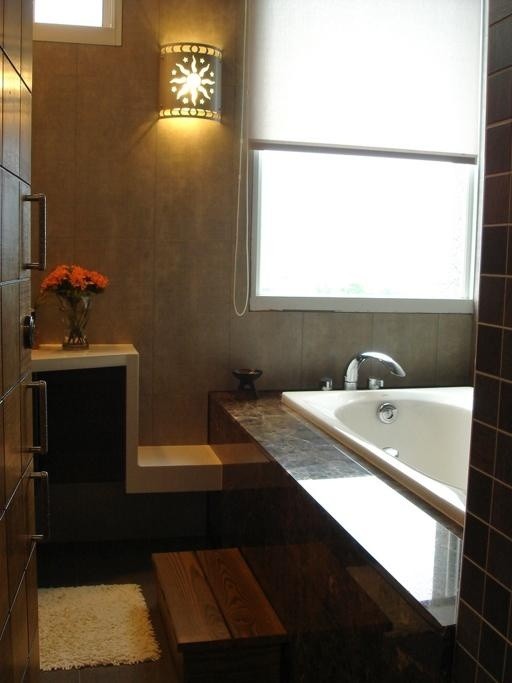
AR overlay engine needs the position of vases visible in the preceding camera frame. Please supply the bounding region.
[58,292,92,349]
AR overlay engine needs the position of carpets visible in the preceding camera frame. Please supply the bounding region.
[36,583,162,673]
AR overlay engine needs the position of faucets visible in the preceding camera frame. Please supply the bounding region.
[342,350,406,391]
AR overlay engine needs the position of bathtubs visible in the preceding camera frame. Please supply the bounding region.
[282,385,473,524]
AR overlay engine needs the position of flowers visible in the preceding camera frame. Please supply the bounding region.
[41,263,109,299]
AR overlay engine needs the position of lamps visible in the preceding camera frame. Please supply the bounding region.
[158,40,224,123]
